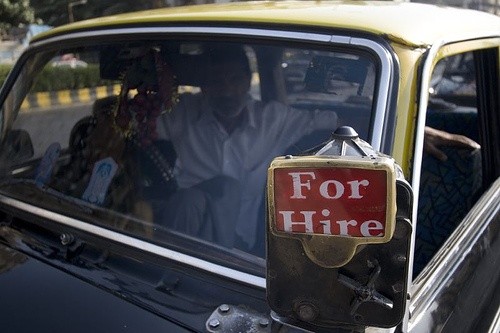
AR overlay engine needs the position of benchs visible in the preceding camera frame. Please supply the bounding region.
[293,100,483,149]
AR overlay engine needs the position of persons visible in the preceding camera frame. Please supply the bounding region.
[71,40,487,261]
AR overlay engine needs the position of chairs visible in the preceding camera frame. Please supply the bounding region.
[403,125,485,284]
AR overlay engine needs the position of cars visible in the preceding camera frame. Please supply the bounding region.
[0,0,500,333]
